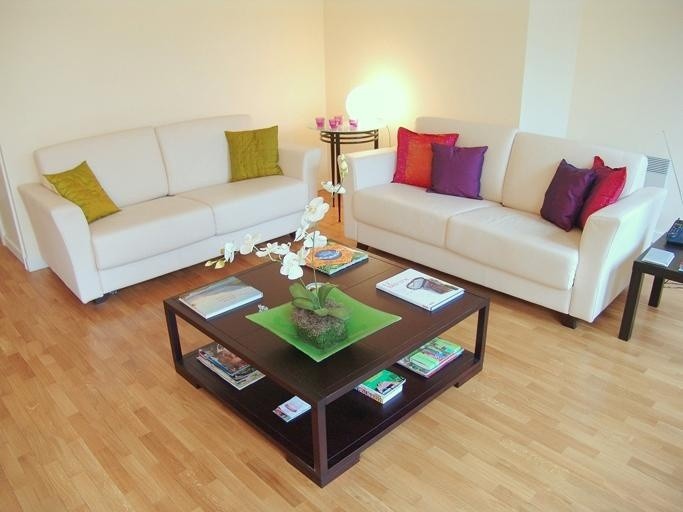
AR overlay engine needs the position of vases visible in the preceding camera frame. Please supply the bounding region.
[245,281,403,364]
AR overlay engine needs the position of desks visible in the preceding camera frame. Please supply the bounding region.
[618,218,682,341]
[305,119,387,223]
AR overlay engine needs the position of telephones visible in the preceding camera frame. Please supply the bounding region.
[666,219,683,246]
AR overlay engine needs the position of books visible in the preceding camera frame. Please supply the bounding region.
[355,383,403,405]
[195,340,266,392]
[374,267,465,312]
[360,368,406,395]
[641,245,675,269]
[271,396,310,423]
[178,275,264,320]
[397,338,463,380]
[296,238,368,275]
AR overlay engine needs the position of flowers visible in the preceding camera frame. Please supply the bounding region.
[204,154,352,320]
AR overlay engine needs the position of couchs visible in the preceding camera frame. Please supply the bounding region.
[337,116,668,329]
[16,113,319,305]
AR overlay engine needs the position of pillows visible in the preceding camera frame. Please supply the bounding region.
[539,156,627,232]
[390,127,488,200]
[40,160,122,224]
[223,124,284,183]
